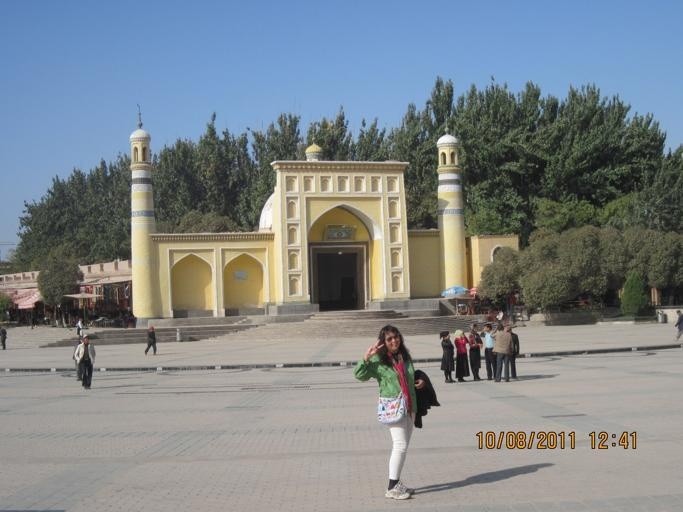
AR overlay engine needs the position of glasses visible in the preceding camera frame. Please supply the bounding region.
[386,335,399,341]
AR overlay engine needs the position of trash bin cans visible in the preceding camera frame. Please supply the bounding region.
[657,311,665,323]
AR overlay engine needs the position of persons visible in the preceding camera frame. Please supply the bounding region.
[7,306,136,330]
[0,327,7,349]
[72,337,83,380]
[439,322,519,381]
[673,310,683,342]
[352,324,425,499]
[144,326,156,355]
[76,317,84,337]
[75,334,96,389]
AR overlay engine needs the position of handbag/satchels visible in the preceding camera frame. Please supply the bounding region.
[378,398,406,424]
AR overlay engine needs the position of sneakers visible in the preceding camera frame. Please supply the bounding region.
[386,481,414,499]
[445,376,517,383]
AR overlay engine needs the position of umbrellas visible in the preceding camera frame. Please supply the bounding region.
[63,292,105,329]
[441,286,478,317]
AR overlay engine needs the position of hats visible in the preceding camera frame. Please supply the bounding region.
[440,331,449,339]
